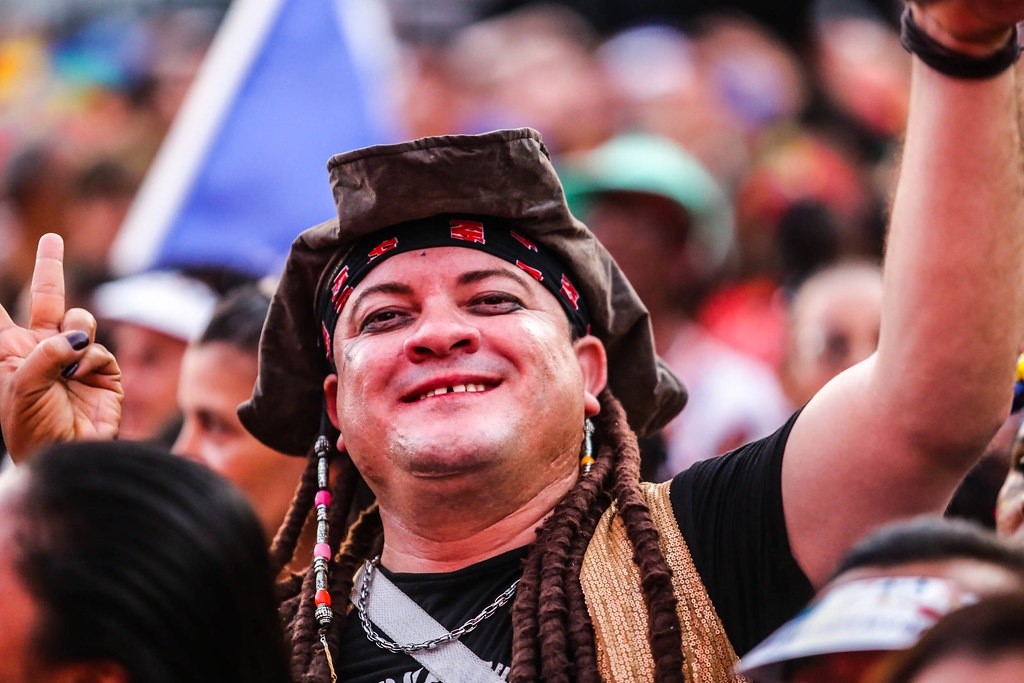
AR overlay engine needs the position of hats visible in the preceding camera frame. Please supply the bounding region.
[95,270,220,344]
[733,559,1024,674]
[237,127,689,458]
[552,130,734,276]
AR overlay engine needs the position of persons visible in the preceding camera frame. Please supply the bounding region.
[0,0,1024,683]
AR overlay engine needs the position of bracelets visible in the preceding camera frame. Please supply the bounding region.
[899,5,1024,81]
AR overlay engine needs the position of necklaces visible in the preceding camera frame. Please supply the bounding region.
[357,417,595,655]
